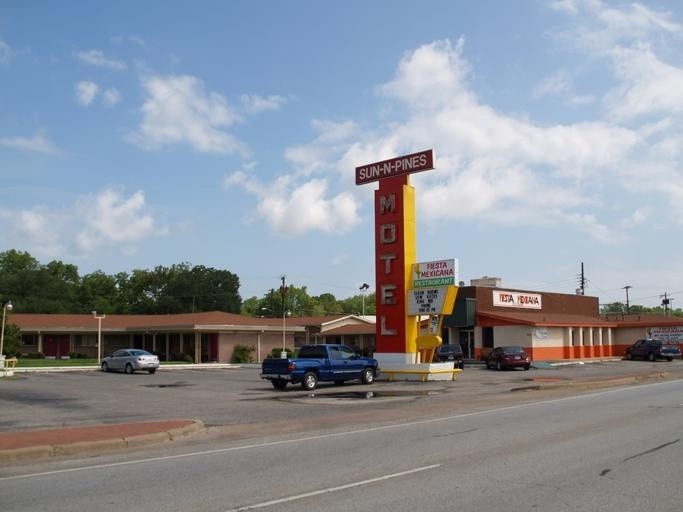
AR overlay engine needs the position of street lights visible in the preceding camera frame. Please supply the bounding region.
[1,300,13,355]
[283,310,291,352]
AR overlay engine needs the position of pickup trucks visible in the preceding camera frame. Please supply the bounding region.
[259,344,380,392]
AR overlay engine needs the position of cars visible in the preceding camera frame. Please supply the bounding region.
[486,346,530,371]
[100,348,160,375]
[625,338,682,362]
[437,343,464,369]
